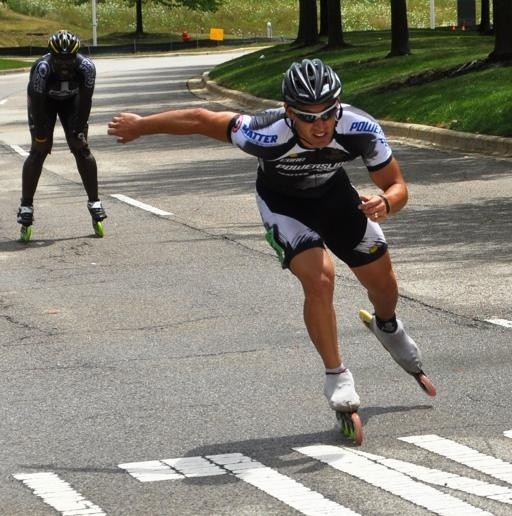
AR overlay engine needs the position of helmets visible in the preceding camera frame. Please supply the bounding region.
[282,58,342,106]
[48,29,81,57]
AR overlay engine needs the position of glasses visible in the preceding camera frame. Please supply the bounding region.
[288,98,339,125]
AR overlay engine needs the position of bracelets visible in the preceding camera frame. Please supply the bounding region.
[377,193,391,215]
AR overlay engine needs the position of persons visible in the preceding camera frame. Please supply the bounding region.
[13,26,108,227]
[103,54,424,415]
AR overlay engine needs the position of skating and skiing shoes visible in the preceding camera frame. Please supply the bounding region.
[16,205,35,243]
[359,309,438,397]
[86,199,107,238]
[322,367,364,446]
